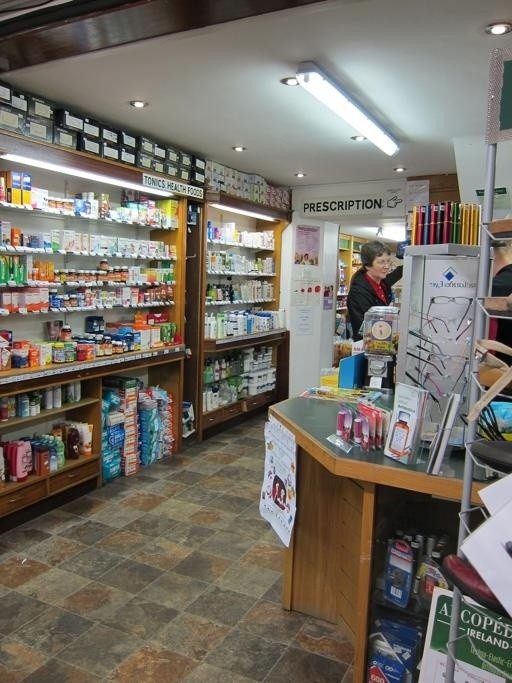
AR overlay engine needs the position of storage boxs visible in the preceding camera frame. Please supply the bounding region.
[134,136,155,156]
[177,152,193,169]
[177,165,191,180]
[163,159,179,177]
[153,158,165,174]
[98,126,119,144]
[10,90,28,112]
[119,131,138,149]
[100,141,119,160]
[191,172,204,185]
[56,109,84,133]
[0,78,13,104]
[53,124,77,150]
[134,151,153,170]
[78,117,100,137]
[164,145,180,163]
[117,144,136,166]
[205,160,267,205]
[192,156,205,175]
[0,102,26,135]
[24,116,53,145]
[154,144,167,161]
[25,93,55,124]
[78,134,101,156]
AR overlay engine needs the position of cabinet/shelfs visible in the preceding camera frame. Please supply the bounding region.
[334,233,368,364]
[199,196,281,340]
[394,243,494,455]
[446,51,511,677]
[197,330,290,442]
[365,596,511,683]
[1,129,184,381]
[0,357,182,525]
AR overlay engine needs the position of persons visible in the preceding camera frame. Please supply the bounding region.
[346,243,403,343]
[301,253,311,266]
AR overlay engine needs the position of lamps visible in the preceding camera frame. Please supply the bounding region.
[295,61,401,157]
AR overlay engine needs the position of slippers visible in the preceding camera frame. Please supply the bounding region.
[487,218,511,239]
[484,264,511,312]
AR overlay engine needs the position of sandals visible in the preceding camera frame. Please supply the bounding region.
[475,338,511,389]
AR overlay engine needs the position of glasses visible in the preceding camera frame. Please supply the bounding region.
[372,259,391,264]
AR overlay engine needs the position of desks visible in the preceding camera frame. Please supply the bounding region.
[269,389,495,682]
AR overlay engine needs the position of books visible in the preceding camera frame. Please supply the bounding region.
[384,383,429,466]
[425,393,462,476]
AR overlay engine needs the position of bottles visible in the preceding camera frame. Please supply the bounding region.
[116,341,124,353]
[64,297,70,305]
[111,341,116,355]
[70,294,78,306]
[54,343,65,363]
[55,436,65,468]
[95,337,104,357]
[74,330,103,339]
[60,325,71,342]
[213,360,220,381]
[49,342,54,362]
[98,258,108,270]
[71,341,77,361]
[65,343,74,363]
[220,359,226,378]
[54,266,128,282]
[104,336,113,355]
[86,340,95,360]
[77,340,86,361]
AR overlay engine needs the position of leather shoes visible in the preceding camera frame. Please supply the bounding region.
[471,439,512,471]
[442,551,511,621]
